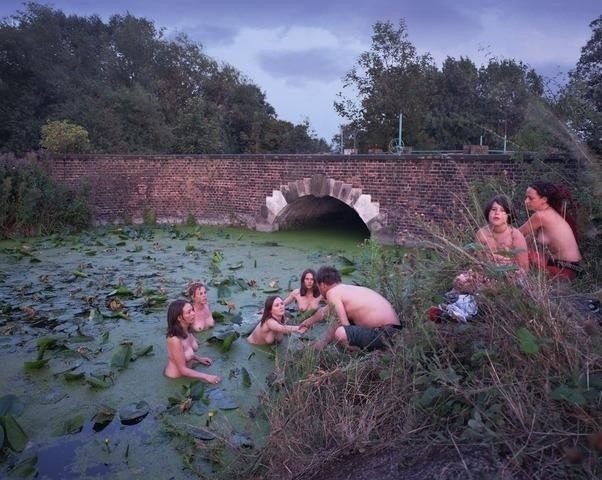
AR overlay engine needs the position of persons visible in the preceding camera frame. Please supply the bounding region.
[189,283,215,332]
[284,269,327,313]
[298,266,401,351]
[247,295,308,344]
[518,182,582,282]
[164,300,223,384]
[452,196,529,295]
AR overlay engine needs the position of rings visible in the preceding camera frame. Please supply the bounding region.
[215,381,216,384]
